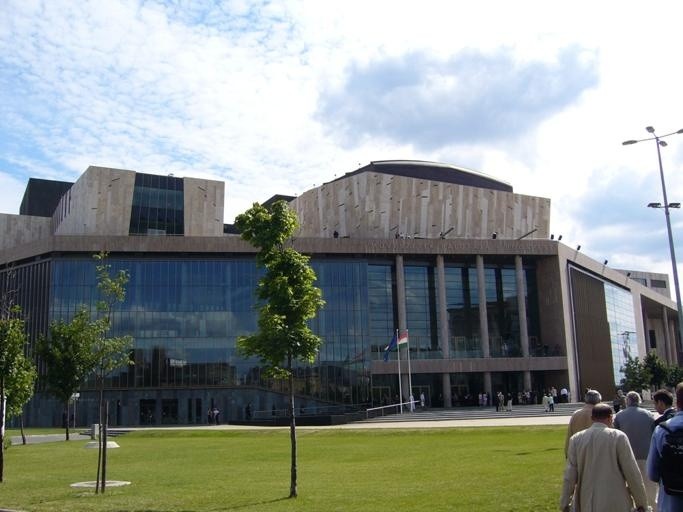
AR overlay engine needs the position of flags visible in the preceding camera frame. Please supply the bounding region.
[382,329,398,362]
[382,328,409,352]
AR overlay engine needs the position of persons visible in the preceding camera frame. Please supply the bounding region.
[564,388,603,461]
[245,399,293,418]
[394,391,425,412]
[559,400,649,512]
[207,408,220,425]
[452,385,567,413]
[651,389,678,433]
[613,390,657,511]
[645,381,683,512]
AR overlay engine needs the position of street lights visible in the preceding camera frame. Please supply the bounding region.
[70,392,80,429]
[620,330,636,366]
[620,125,683,352]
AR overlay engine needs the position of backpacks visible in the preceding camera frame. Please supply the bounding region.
[658,421,682,496]
